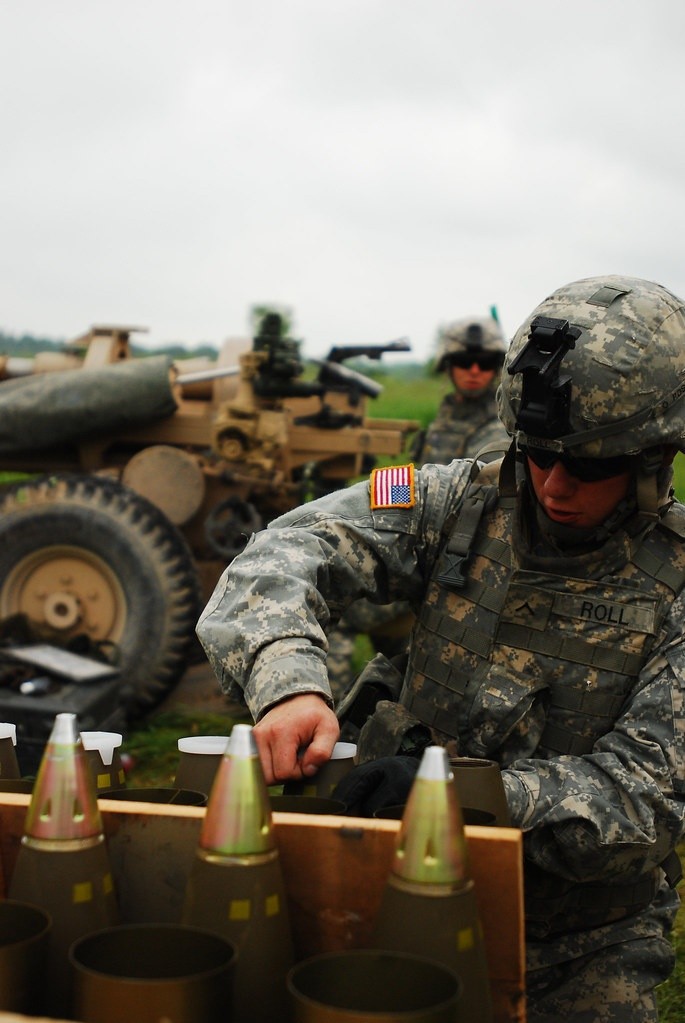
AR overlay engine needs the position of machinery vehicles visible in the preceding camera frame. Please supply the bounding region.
[0,322,426,765]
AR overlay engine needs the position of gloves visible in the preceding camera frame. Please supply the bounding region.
[331,757,421,819]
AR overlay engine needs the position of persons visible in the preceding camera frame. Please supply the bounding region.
[418,314,513,469]
[195,275,685,1023]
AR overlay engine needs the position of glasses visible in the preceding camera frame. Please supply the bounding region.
[515,440,641,484]
[454,354,496,371]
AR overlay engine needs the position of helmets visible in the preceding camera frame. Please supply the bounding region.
[495,281,684,456]
[259,313,287,336]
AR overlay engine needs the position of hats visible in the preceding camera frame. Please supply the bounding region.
[435,324,506,374]
